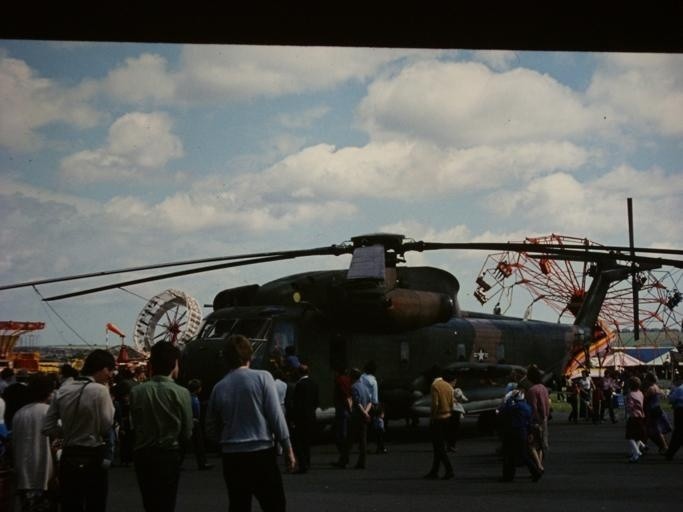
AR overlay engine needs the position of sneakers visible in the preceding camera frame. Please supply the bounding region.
[531,469,542,482]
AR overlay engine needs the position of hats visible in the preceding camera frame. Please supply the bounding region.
[512,383,518,388]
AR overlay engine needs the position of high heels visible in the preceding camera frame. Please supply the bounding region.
[629,452,643,464]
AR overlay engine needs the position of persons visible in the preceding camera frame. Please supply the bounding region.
[527,365,550,476]
[177,379,208,472]
[265,359,289,450]
[292,365,321,442]
[42,348,116,512]
[446,375,466,452]
[13,375,62,512]
[551,374,565,403]
[206,336,296,512]
[117,362,140,469]
[505,368,527,391]
[581,371,596,417]
[668,373,683,441]
[283,344,303,375]
[330,368,373,470]
[3,371,30,431]
[625,375,645,464]
[332,365,353,468]
[565,376,582,424]
[354,367,379,454]
[134,364,147,387]
[59,358,78,380]
[599,371,619,424]
[129,341,192,511]
[424,366,456,482]
[0,367,16,388]
[378,411,389,455]
[495,388,544,481]
[645,373,672,454]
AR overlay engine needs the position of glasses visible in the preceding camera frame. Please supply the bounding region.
[673,379,679,382]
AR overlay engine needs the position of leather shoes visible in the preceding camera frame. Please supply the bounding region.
[354,463,367,469]
[330,460,346,468]
[424,471,438,479]
[198,462,211,470]
[441,472,455,480]
